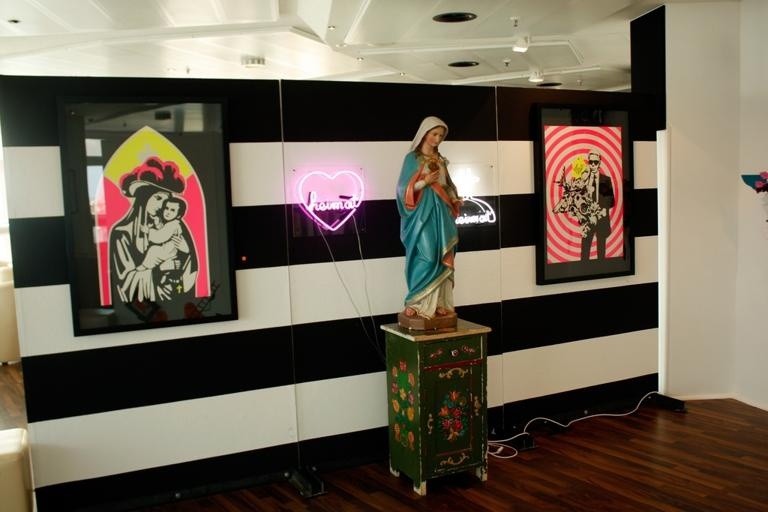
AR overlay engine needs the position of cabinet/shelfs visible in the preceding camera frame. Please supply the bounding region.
[380,319,494,498]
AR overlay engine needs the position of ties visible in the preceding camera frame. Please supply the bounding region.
[592,176,597,202]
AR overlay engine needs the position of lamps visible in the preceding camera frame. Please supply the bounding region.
[527,62,544,84]
[510,33,529,54]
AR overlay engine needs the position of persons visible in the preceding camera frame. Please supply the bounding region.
[106,184,197,299]
[394,114,463,319]
[579,149,614,259]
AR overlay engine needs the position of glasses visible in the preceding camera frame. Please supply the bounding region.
[589,160,598,165]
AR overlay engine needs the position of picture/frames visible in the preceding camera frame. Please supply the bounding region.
[530,101,637,287]
[56,96,240,340]
